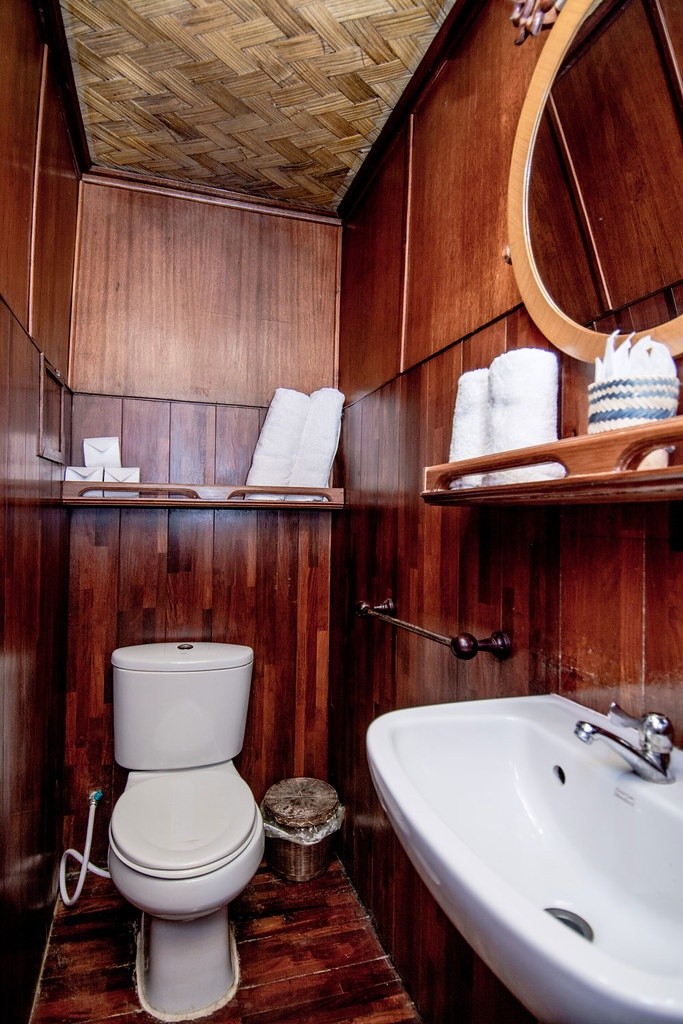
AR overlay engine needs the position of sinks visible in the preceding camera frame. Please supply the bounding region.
[362,692,680,1023]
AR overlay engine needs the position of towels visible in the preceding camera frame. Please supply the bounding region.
[482,347,560,490]
[281,388,348,506]
[448,366,492,494]
[245,386,313,501]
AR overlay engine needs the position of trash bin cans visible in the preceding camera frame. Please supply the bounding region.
[259,776,340,882]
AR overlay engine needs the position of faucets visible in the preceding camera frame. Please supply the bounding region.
[573,702,678,785]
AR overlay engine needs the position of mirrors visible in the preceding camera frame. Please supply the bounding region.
[506,0,683,367]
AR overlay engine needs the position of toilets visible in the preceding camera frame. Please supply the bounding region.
[106,641,268,1024]
